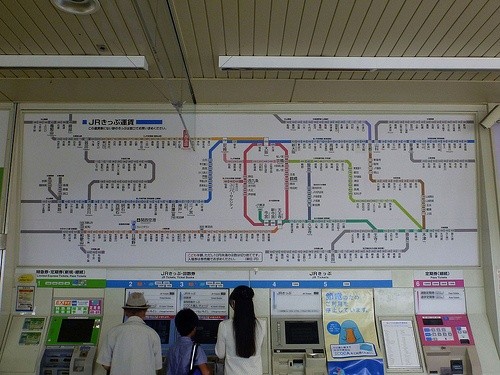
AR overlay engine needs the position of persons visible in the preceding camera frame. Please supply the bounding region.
[96,292,163,375]
[164,308,212,375]
[214,284,267,375]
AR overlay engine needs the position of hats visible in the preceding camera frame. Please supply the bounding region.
[121,292,149,308]
